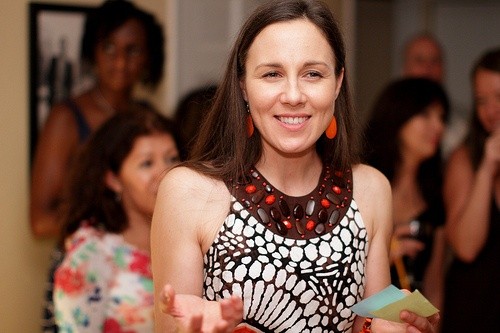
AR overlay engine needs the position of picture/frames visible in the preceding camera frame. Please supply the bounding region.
[29,2,92,169]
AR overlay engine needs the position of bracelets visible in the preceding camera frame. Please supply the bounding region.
[361,316,373,333]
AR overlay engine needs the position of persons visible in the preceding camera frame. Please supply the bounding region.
[29,0,165,333]
[443,48,500,333]
[358,75,451,332]
[150,0,440,332]
[52,107,180,333]
[405,35,444,82]
[48,36,74,109]
